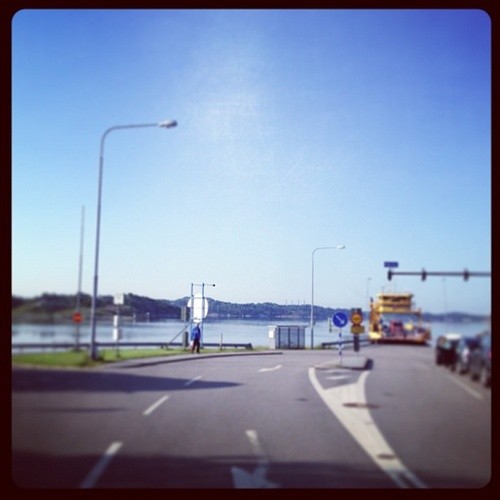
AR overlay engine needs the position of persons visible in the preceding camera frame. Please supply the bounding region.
[190,322,203,354]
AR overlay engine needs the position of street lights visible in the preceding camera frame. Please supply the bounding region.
[309,245,346,349]
[87,119,179,365]
